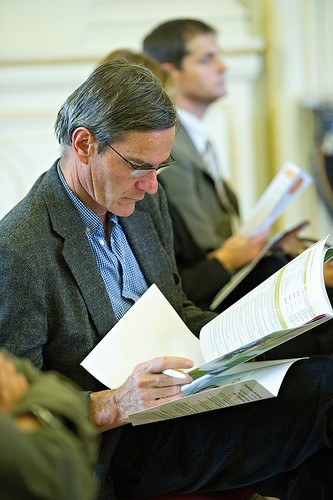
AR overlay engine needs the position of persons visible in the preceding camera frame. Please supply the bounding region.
[0,19,333,500]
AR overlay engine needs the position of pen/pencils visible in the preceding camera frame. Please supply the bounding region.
[162,368,188,381]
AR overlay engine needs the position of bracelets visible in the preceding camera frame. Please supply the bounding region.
[32,404,64,431]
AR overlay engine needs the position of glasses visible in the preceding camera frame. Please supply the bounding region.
[104,141,175,177]
